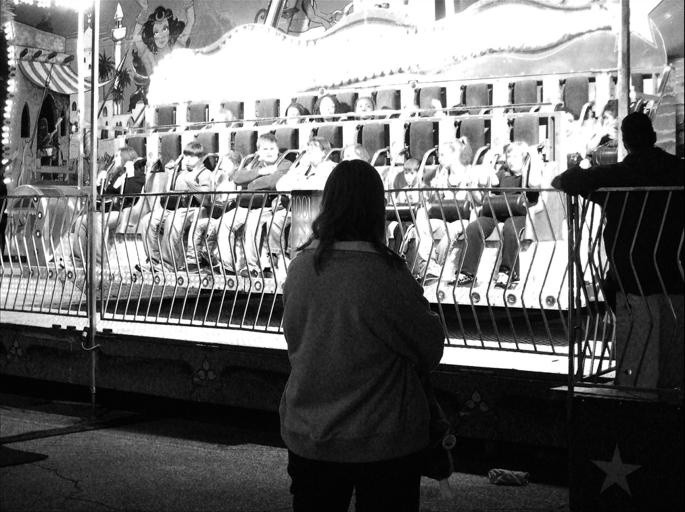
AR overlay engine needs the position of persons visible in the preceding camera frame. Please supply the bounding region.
[552,110,685,389]
[133,0,196,76]
[69,94,542,289]
[277,159,444,510]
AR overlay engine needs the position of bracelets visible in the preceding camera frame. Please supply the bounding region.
[182,1,194,12]
[135,10,148,26]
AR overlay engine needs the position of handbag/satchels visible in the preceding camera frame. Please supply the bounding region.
[420,380,455,481]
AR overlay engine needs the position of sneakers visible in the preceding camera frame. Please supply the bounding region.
[494,272,518,290]
[416,274,442,286]
[447,272,478,288]
[136,261,274,277]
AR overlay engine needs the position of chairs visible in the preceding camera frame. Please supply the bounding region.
[113,74,596,244]
